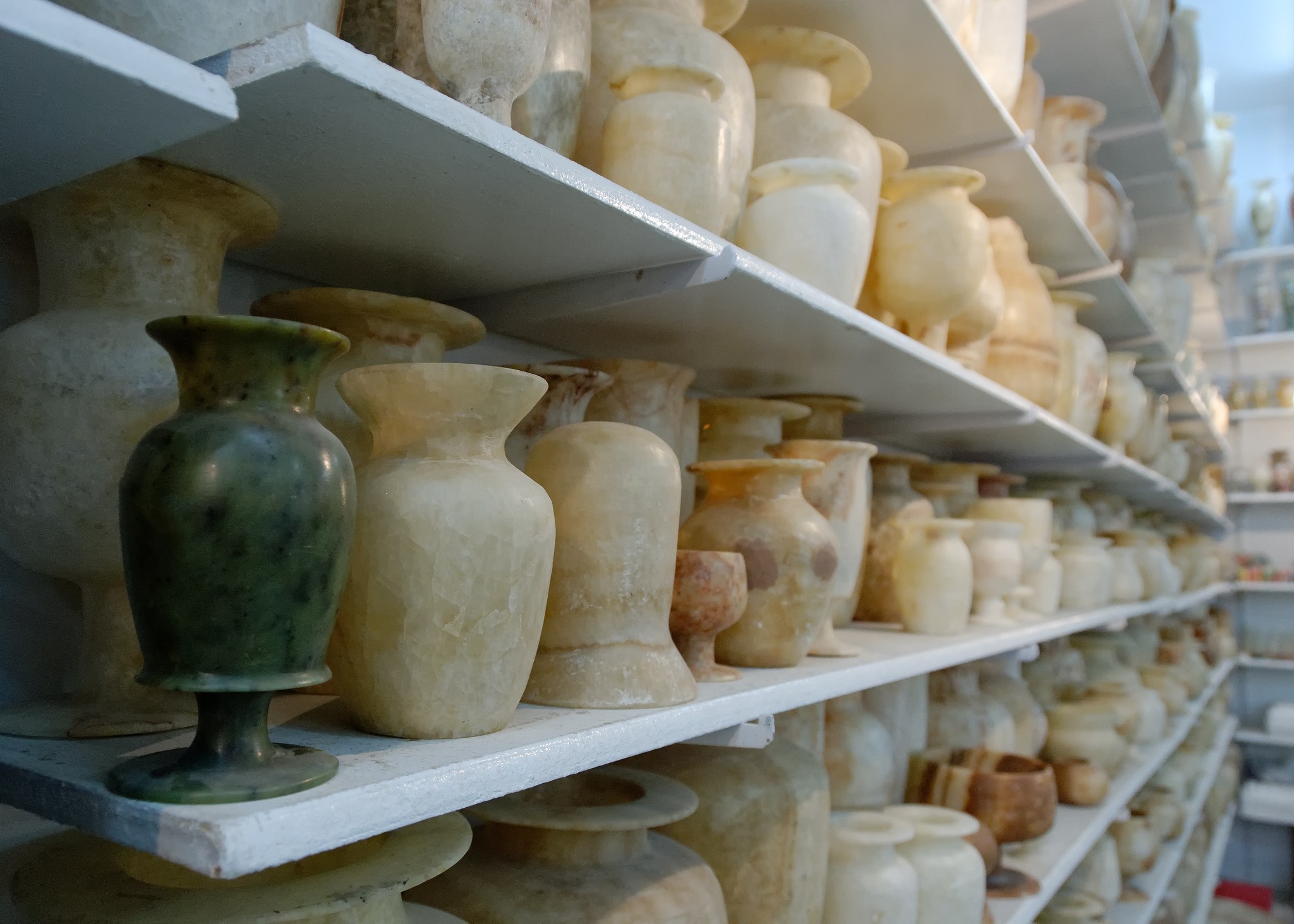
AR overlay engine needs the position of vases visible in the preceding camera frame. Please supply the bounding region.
[1,0,1294,923]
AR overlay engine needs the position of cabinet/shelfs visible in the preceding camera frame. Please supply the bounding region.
[1221,242,1293,827]
[1,1,1240,924]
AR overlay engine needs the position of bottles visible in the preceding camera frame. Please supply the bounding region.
[0,3,1290,923]
